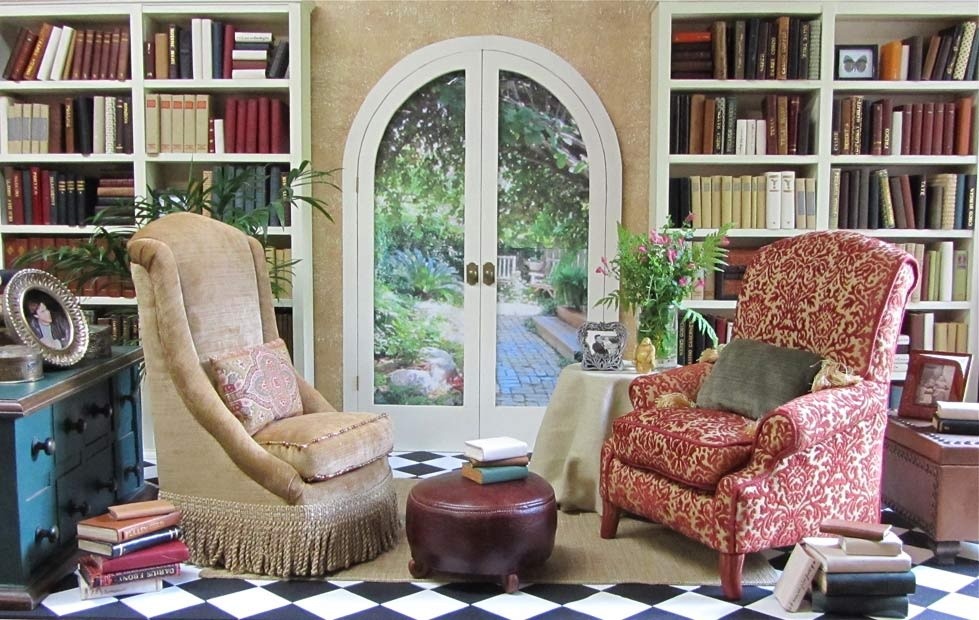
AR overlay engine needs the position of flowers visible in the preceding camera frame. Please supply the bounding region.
[594,212,733,358]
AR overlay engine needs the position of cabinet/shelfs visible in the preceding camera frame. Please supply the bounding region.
[0,346,146,611]
[649,1,979,405]
[0,1,315,391]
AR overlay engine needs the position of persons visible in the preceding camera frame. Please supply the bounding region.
[593,335,613,354]
[27,302,67,350]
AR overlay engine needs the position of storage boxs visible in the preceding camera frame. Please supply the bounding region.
[879,412,979,566]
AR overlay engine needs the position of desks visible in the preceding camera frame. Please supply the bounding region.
[530,361,687,511]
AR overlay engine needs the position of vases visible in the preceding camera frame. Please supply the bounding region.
[640,304,678,368]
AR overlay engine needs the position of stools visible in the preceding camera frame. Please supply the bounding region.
[404,469,557,593]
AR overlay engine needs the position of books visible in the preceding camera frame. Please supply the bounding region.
[144,18,289,80]
[152,164,294,366]
[0,95,132,154]
[83,309,139,346]
[671,16,979,81]
[2,24,130,81]
[146,94,290,153]
[462,435,529,484]
[669,168,977,230]
[890,313,968,380]
[774,519,916,619]
[6,237,136,298]
[670,92,974,155]
[932,400,979,436]
[77,499,189,600]
[677,241,760,366]
[3,166,135,225]
[888,241,968,302]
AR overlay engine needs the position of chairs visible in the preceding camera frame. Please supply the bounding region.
[123,211,402,579]
[599,230,912,599]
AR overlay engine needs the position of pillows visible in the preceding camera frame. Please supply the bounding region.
[697,338,821,419]
[210,340,303,435]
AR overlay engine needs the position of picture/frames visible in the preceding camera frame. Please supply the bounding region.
[834,44,879,79]
[2,268,90,366]
[580,321,630,370]
